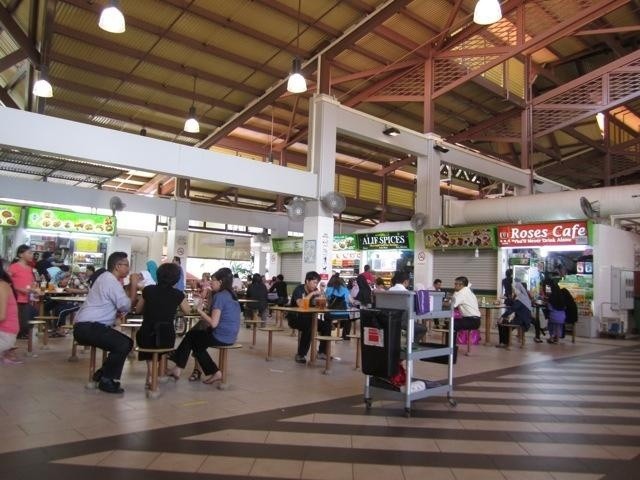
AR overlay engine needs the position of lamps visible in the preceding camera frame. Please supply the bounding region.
[383,125,401,138]
[183,68,201,136]
[474,0,503,27]
[288,0,308,96]
[99,0,127,34]
[433,141,450,154]
[532,177,545,186]
[31,9,55,98]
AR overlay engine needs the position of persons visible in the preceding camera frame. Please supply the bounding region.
[234,266,482,362]
[497,269,579,348]
[1,243,95,365]
[73,252,242,394]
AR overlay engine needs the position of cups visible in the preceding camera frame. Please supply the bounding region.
[316,297,330,309]
[302,298,310,310]
[33,281,54,292]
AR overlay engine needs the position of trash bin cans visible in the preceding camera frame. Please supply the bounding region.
[360,308,402,377]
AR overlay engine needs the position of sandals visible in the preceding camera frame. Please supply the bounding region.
[94,368,223,393]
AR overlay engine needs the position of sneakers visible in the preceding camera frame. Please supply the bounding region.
[319,353,340,361]
[296,354,306,363]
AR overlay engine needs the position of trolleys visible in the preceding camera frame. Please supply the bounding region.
[359,289,456,417]
[599,301,626,340]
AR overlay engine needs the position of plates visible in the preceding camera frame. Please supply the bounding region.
[332,237,355,250]
[40,209,114,233]
[433,227,491,248]
[0,210,18,225]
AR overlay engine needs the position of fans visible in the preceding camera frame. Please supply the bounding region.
[408,213,428,231]
[579,196,601,218]
[321,191,348,216]
[109,196,127,216]
[286,200,309,222]
